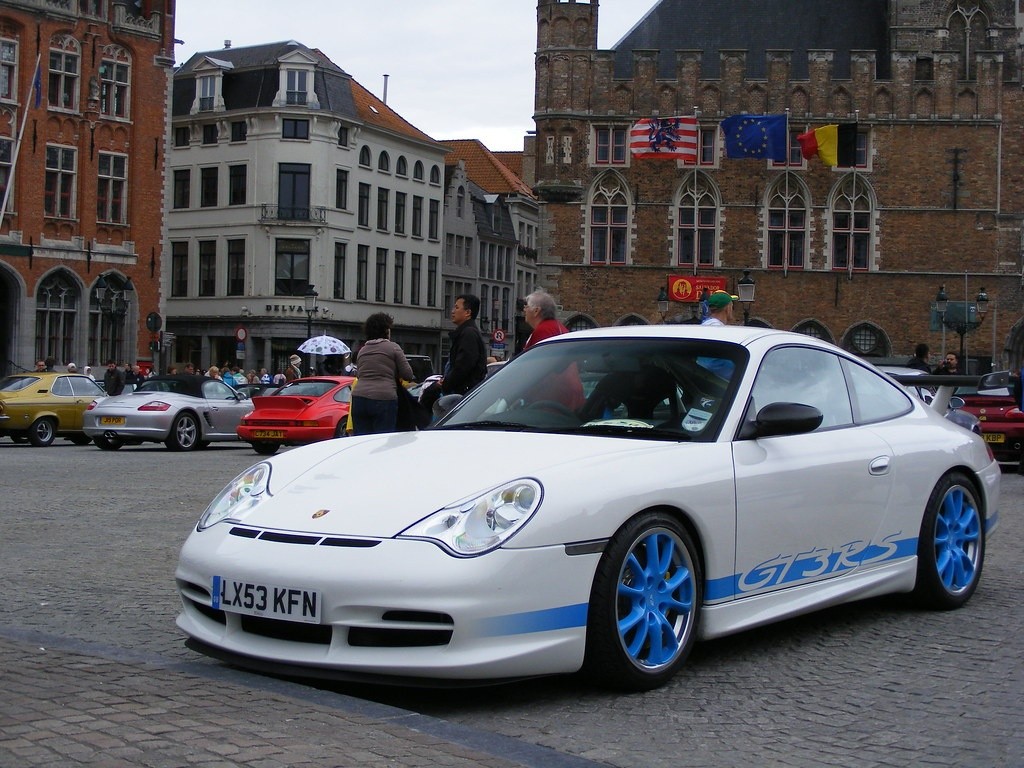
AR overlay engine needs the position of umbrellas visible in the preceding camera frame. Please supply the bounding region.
[297,329,352,363]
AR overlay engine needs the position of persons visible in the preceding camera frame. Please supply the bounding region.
[399,349,501,430]
[432,294,488,423]
[933,351,966,375]
[352,313,414,436]
[695,290,739,380]
[905,343,936,396]
[521,286,585,416]
[34,354,357,396]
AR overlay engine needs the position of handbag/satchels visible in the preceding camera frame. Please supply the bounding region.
[279,374,284,386]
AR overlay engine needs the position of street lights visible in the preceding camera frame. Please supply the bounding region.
[301,284,318,377]
[95,274,134,365]
[936,282,990,371]
[736,270,756,327]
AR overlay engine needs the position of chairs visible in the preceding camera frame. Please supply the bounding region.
[752,369,821,408]
[581,369,686,427]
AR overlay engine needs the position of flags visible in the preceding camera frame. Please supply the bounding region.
[797,122,858,166]
[629,114,697,160]
[720,113,788,160]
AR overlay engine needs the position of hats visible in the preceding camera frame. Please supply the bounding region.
[68,363,76,369]
[290,355,302,364]
[708,290,739,308]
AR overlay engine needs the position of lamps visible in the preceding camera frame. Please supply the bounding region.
[242,307,248,311]
[323,307,329,312]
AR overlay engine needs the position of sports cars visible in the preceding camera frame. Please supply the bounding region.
[175,325,1001,690]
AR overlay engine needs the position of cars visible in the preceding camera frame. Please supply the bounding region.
[876,364,981,436]
[232,383,281,400]
[0,372,109,446]
[952,376,1024,471]
[82,375,256,453]
[237,375,358,454]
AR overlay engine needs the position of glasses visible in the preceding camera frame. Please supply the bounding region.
[38,365,44,366]
